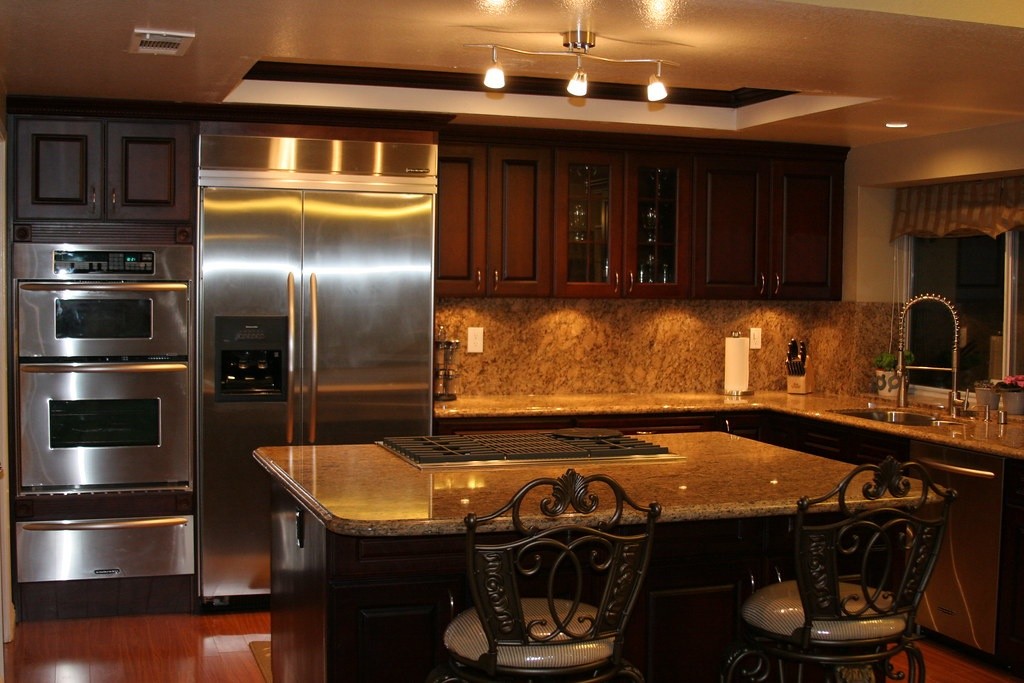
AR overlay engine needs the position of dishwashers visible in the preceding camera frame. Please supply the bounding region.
[907,437,1006,655]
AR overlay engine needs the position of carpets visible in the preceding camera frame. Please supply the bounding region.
[249,640,272,683]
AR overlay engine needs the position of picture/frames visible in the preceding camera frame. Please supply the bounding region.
[1001,391,1024,415]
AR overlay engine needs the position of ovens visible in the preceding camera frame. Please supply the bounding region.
[12,242,194,583]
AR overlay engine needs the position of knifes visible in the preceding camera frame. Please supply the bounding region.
[786,338,806,376]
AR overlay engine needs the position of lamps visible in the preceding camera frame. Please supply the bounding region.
[647,61,667,101]
[433,320,460,400]
[484,47,505,89]
[566,56,588,96]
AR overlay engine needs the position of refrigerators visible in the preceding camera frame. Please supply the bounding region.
[194,135,438,606]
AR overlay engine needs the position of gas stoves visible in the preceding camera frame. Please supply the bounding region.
[375,428,687,469]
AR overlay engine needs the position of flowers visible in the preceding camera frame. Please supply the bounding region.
[998,374,1024,391]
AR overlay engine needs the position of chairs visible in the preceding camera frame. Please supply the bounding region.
[718,456,958,683]
[426,469,661,683]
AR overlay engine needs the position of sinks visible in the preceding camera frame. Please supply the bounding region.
[822,408,969,427]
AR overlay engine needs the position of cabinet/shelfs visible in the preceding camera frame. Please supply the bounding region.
[555,145,694,300]
[772,419,1024,671]
[437,144,553,297]
[7,113,192,225]
[696,147,845,300]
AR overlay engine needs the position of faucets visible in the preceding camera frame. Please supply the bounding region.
[895,293,961,392]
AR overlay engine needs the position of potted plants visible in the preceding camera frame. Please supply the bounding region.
[974,380,1000,409]
[872,350,914,397]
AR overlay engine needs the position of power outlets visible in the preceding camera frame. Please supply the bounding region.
[467,327,483,353]
[749,327,761,349]
